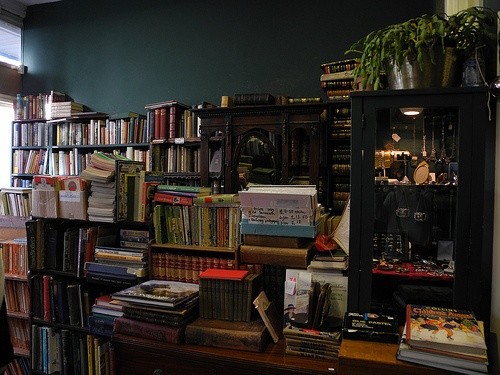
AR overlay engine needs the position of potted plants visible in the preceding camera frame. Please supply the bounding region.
[342,5,500,91]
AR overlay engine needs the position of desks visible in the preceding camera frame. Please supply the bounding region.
[108,319,339,375]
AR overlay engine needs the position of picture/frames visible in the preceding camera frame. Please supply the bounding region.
[253,290,282,344]
[114,158,144,222]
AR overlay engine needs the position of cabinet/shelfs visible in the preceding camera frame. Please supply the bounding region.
[0,86,500,375]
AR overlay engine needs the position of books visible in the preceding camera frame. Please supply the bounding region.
[0,58,488,375]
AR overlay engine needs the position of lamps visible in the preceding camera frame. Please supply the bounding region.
[399,107,423,116]
